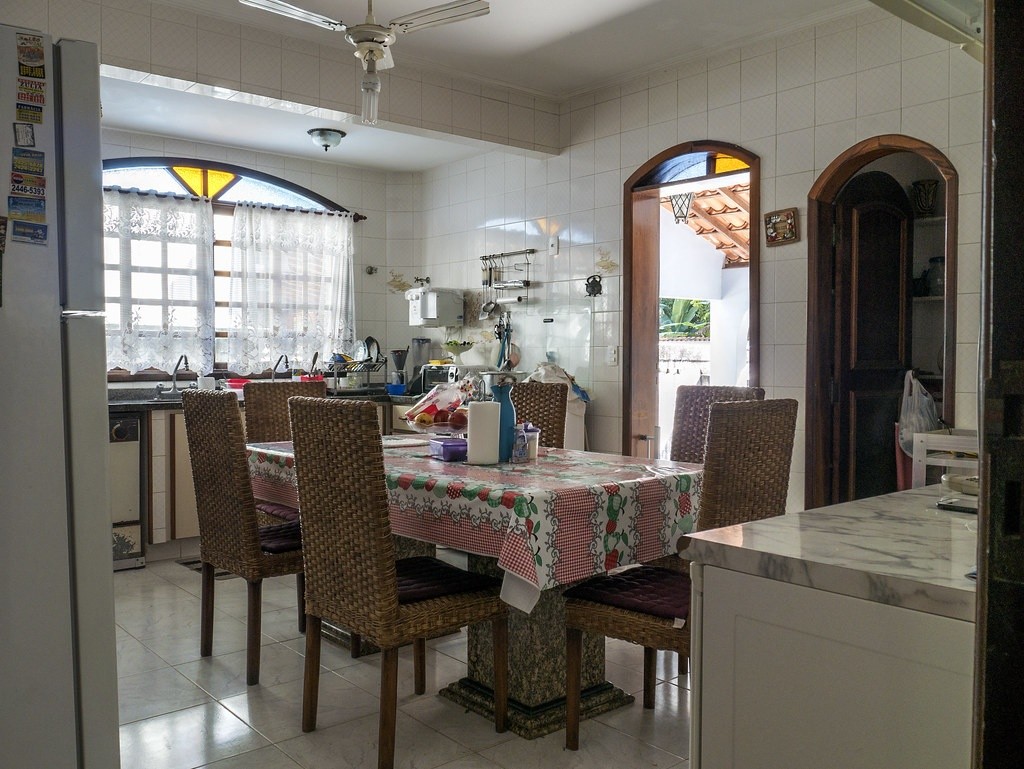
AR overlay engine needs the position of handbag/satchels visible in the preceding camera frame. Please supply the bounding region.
[899,370,939,458]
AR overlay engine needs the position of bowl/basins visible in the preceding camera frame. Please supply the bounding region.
[385,384,406,395]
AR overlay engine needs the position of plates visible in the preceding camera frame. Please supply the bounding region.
[329,336,380,372]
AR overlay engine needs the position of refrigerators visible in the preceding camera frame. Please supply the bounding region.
[0,23,122,769]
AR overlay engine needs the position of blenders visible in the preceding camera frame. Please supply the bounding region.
[388,345,410,384]
[408,335,432,396]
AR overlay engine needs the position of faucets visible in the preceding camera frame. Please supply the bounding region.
[169,356,190,392]
[271,354,289,380]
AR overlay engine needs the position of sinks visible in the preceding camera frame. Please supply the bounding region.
[147,399,184,404]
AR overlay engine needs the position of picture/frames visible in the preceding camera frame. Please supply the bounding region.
[764,207,802,247]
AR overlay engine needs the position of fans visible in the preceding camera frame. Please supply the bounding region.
[239,0,489,71]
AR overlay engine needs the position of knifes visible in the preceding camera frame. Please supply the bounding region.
[309,351,318,377]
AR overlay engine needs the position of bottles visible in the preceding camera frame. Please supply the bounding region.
[511,423,529,463]
[923,255,944,296]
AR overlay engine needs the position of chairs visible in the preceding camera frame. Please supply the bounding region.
[242,380,327,444]
[642,383,765,709]
[286,394,512,769]
[560,398,799,753]
[496,382,568,449]
[181,389,307,687]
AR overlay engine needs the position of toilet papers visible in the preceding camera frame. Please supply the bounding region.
[467,401,501,465]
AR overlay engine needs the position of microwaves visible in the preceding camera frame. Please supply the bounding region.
[421,364,490,396]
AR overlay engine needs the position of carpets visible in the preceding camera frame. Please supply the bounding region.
[174,555,243,581]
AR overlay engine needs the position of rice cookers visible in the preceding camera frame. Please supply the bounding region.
[478,370,526,401]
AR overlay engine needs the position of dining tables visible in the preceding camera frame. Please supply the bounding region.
[246,432,707,742]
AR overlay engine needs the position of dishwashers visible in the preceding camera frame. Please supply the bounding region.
[108,412,145,572]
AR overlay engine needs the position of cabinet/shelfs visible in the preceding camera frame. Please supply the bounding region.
[678,475,980,769]
[911,216,947,402]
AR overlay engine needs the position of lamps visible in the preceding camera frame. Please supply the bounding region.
[670,192,695,224]
[354,41,387,125]
